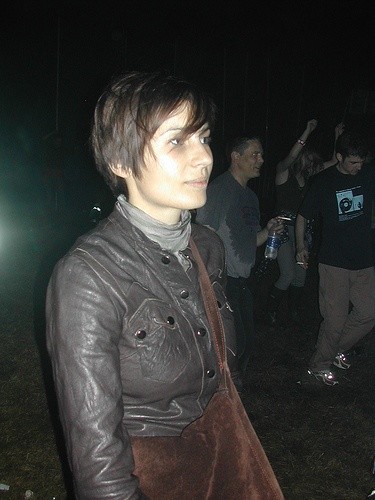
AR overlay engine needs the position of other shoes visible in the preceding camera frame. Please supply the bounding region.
[333,352,352,369]
[308,367,339,385]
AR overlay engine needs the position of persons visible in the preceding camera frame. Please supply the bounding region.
[44,69,284,499]
[192,131,285,388]
[294,129,375,386]
[261,118,346,329]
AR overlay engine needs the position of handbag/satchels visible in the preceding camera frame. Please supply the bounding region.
[127,370,286,500]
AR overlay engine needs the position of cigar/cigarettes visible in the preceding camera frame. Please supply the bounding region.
[296,260,306,264]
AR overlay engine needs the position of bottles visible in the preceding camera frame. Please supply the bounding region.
[264,219,280,259]
